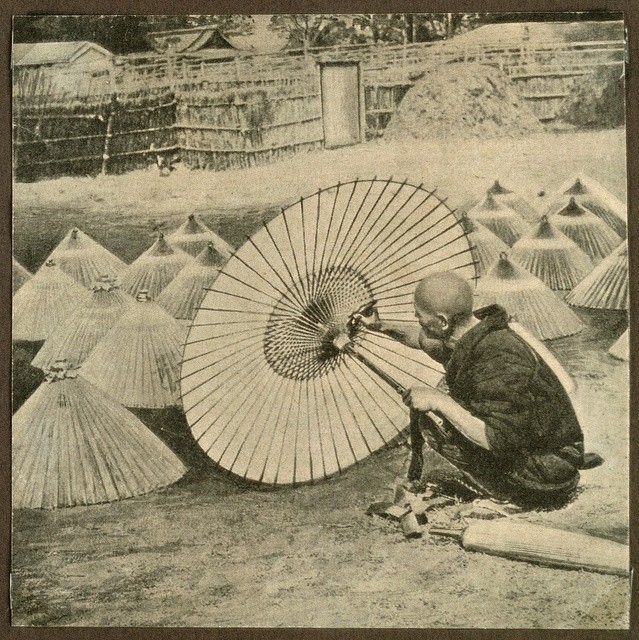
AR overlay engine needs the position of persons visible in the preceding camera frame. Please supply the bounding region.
[348,272,584,515]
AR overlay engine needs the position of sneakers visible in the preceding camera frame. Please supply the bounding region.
[456,474,512,504]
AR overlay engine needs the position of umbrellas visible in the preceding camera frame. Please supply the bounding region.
[565,237,630,311]
[509,214,594,290]
[42,227,128,291]
[154,240,230,321]
[503,312,576,395]
[31,273,136,370]
[12,258,89,341]
[173,173,480,486]
[77,289,188,409]
[478,180,541,230]
[475,251,584,340]
[554,178,627,238]
[466,193,532,248]
[12,357,187,508]
[116,232,196,301]
[168,214,235,264]
[458,210,510,277]
[548,197,624,267]
